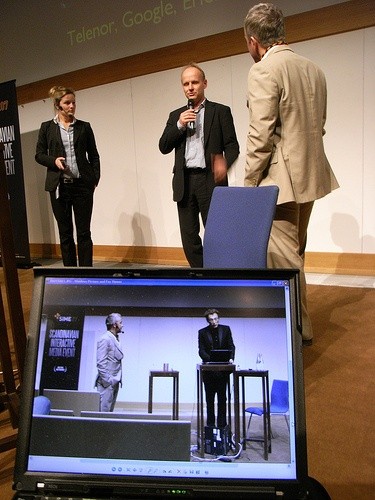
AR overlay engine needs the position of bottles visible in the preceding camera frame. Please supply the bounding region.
[255,354,264,371]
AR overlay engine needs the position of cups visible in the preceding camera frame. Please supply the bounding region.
[163,363,168,371]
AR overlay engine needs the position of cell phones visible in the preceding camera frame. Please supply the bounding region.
[61,160,67,168]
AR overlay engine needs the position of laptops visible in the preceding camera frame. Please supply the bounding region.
[207,349,231,364]
[11,267,326,500]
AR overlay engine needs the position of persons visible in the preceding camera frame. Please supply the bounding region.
[158,65,240,269]
[35,85,100,267]
[198,309,236,427]
[93,313,124,411]
[244,2,340,346]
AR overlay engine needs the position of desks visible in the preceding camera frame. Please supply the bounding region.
[236,369,272,461]
[197,364,236,455]
[148,370,179,420]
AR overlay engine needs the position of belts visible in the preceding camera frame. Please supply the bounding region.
[58,178,84,184]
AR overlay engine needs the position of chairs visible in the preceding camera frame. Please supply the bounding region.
[244,379,290,439]
[202,186,280,267]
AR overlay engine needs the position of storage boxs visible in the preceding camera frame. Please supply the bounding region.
[203,425,233,456]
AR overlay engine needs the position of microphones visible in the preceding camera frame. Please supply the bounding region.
[121,331,124,333]
[58,106,73,120]
[188,99,194,130]
